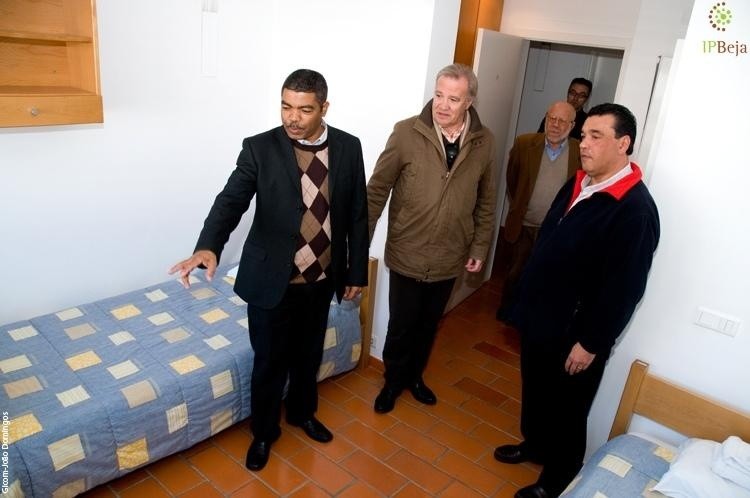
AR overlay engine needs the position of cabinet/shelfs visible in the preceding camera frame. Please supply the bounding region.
[0,0,105,128]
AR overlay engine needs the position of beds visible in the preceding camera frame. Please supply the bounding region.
[560,360,750,498]
[0,256,377,498]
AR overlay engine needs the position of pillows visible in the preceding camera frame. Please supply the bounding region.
[650,434,750,497]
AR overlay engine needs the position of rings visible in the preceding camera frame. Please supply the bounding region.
[577,366,583,370]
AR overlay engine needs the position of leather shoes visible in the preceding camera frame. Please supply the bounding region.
[404,381,436,405]
[514,483,557,498]
[494,444,542,465]
[374,383,400,413]
[285,414,333,443]
[246,426,281,471]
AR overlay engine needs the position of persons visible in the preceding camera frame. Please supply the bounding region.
[168,68,370,471]
[537,77,592,141]
[493,101,661,497]
[503,101,582,277]
[366,62,498,414]
